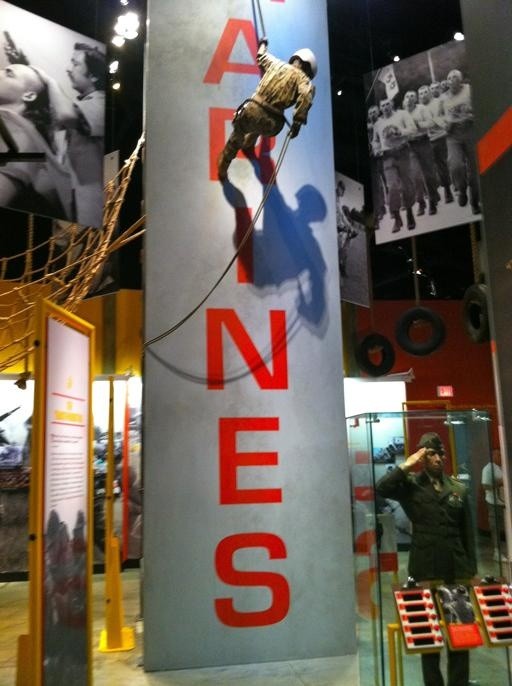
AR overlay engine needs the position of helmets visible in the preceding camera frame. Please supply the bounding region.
[288,48,318,79]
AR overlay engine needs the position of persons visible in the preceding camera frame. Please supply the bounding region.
[335,180,359,276]
[364,68,482,234]
[436,585,476,626]
[1,29,107,229]
[215,35,319,180]
[374,430,486,686]
[480,448,509,565]
[40,506,91,685]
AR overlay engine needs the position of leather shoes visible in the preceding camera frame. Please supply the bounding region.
[391,216,402,233]
[404,211,416,230]
[470,197,480,213]
[443,185,453,203]
[415,200,425,214]
[458,186,468,207]
[426,197,437,215]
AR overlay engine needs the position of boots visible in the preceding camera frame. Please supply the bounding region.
[216,150,231,179]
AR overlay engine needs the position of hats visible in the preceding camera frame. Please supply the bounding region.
[416,430,447,454]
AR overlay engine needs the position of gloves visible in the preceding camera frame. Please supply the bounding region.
[286,121,301,138]
[258,36,270,46]
